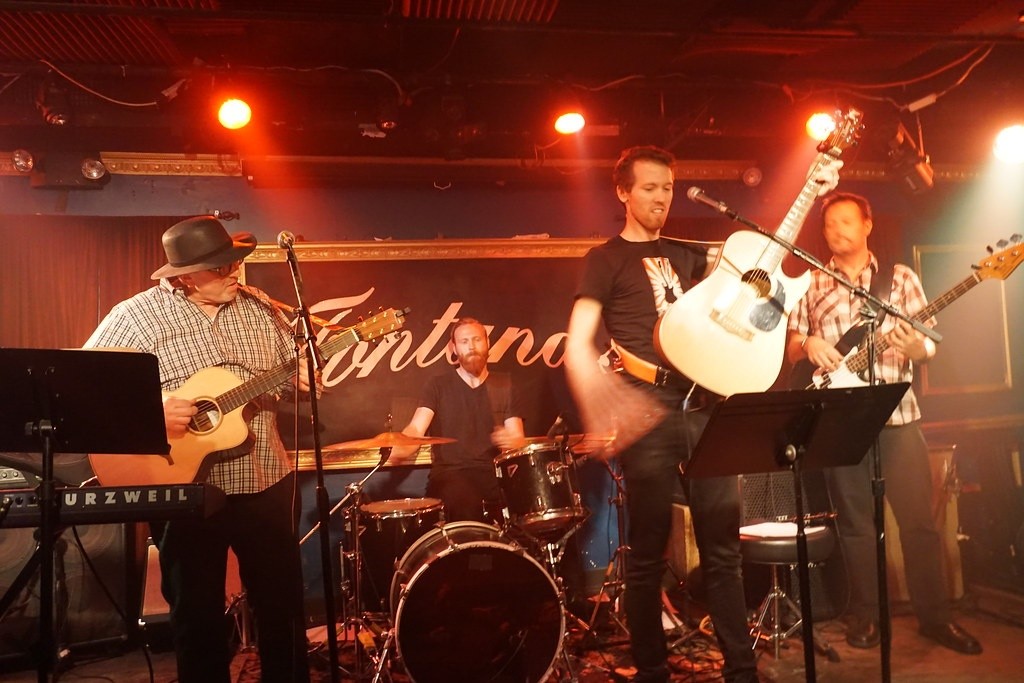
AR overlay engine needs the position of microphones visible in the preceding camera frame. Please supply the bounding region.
[687,186,736,217]
[547,411,567,439]
[277,230,295,249]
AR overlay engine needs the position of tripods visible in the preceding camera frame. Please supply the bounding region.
[298,445,393,683]
[576,459,689,657]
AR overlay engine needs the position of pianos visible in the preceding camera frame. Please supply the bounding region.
[0,484,229,683]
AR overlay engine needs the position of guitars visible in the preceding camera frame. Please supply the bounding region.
[653,106,866,415]
[791,231,1024,391]
[87,304,411,486]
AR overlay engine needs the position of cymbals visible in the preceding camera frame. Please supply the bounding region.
[319,432,459,451]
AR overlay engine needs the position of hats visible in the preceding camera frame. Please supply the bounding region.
[151,216,258,279]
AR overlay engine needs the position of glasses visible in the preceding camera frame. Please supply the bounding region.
[207,258,244,277]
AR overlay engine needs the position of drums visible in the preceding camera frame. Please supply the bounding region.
[348,498,445,623]
[388,520,566,682]
[491,440,587,534]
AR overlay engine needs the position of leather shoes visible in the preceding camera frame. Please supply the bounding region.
[919,616,982,655]
[847,609,881,647]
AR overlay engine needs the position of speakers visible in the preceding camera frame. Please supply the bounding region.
[138,535,252,654]
[734,470,850,620]
[0,452,139,673]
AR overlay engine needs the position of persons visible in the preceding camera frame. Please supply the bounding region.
[562,146,761,683]
[390,317,523,519]
[80,214,317,683]
[783,194,984,655]
